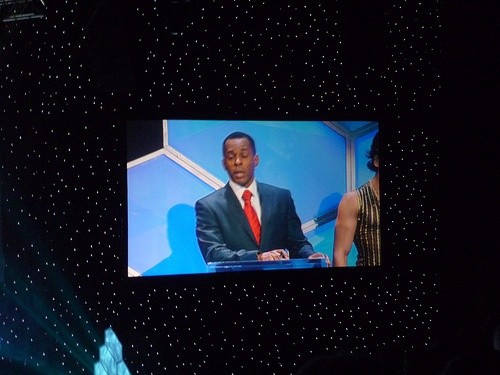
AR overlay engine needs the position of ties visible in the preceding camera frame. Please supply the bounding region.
[241,190,261,246]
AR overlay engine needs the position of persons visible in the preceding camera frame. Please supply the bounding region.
[195,132,330,267]
[332,131,380,267]
